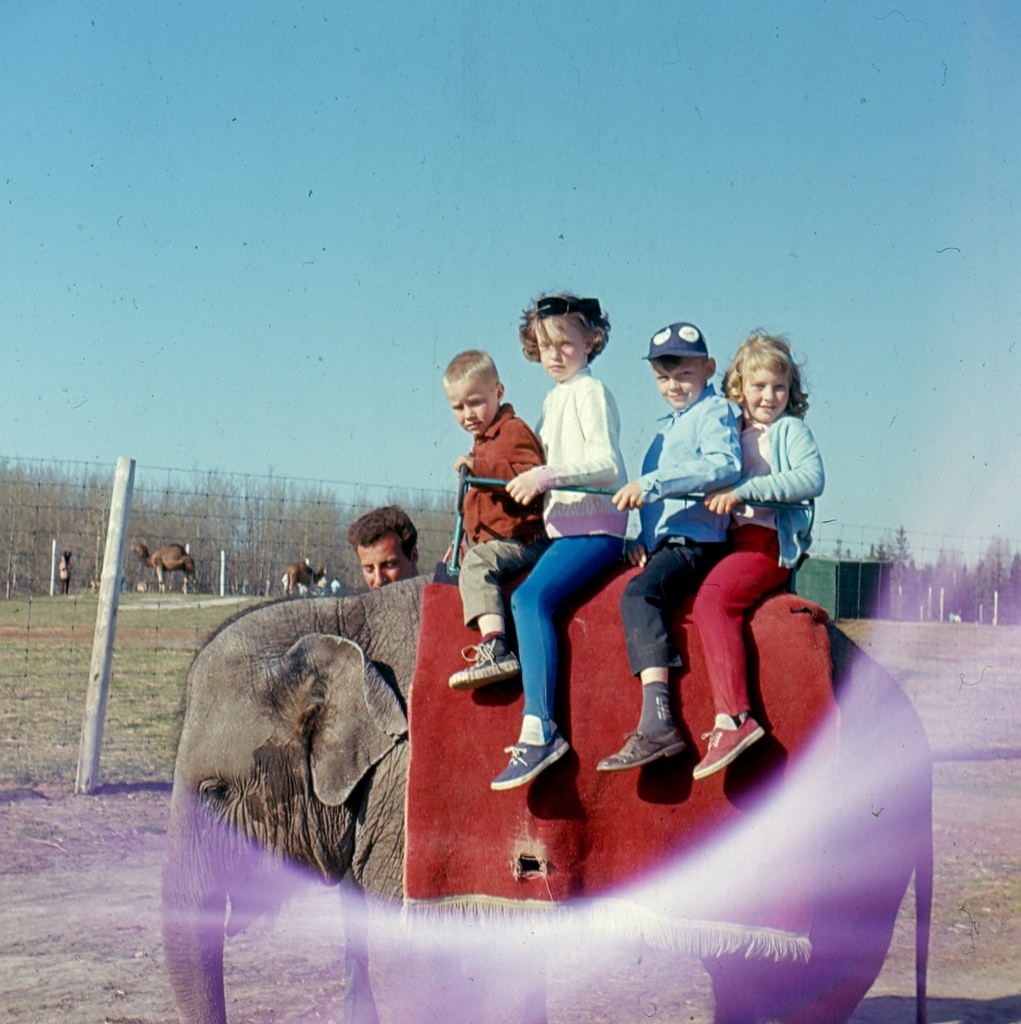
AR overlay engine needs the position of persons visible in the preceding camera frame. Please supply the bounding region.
[337,503,420,1024]
[58,555,68,595]
[442,292,826,794]
[314,561,342,597]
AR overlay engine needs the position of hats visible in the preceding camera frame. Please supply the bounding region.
[647,322,708,361]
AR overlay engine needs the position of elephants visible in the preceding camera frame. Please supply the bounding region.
[160,567,936,1024]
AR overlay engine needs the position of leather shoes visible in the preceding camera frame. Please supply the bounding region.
[596,725,685,772]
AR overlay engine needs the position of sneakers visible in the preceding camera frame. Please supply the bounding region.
[490,729,570,794]
[692,716,765,782]
[449,633,521,689]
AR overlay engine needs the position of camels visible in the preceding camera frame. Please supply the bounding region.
[283,560,324,597]
[130,542,199,595]
